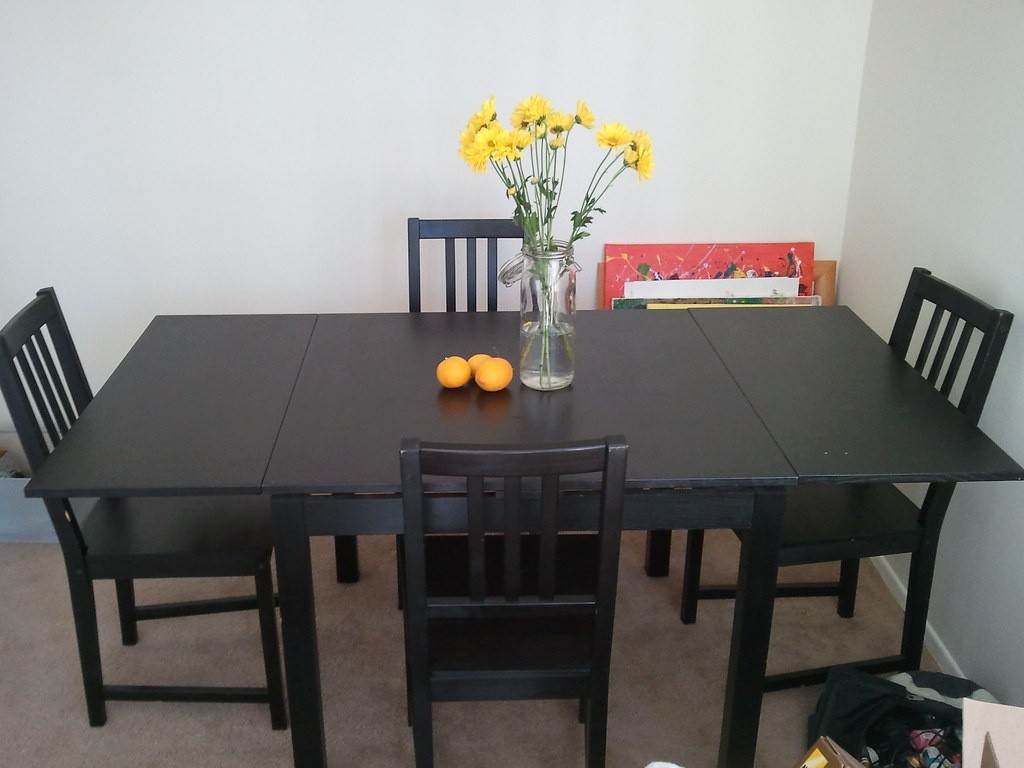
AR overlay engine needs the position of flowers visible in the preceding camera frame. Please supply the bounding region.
[457,92,654,388]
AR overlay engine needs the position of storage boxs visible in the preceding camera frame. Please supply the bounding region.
[0,450,101,544]
[793,731,1000,768]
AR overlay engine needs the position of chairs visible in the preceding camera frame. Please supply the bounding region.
[0,287,289,730]
[399,434,629,768]
[396,219,545,612]
[680,267,1014,768]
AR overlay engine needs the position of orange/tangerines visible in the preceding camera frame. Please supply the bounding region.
[436,354,513,391]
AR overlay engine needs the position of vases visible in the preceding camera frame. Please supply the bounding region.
[498,241,578,390]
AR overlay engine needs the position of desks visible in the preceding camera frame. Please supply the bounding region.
[25,306,1024,768]
